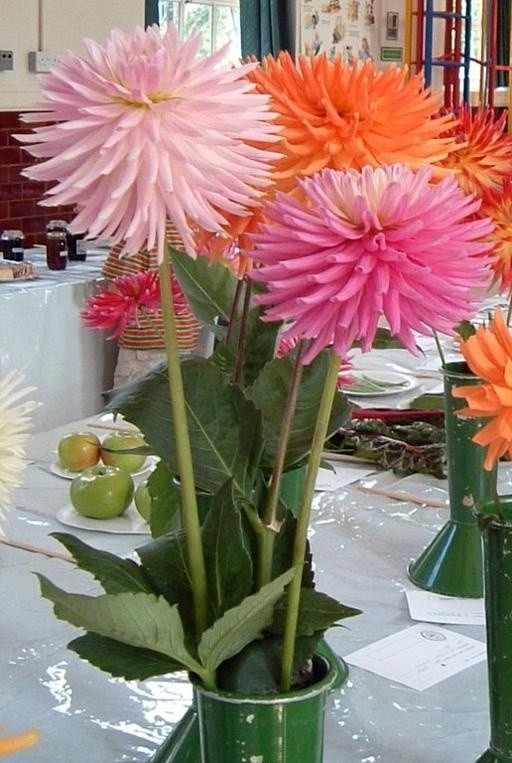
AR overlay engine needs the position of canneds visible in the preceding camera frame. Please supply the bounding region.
[46,219,87,270]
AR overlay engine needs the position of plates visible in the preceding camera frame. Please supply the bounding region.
[51,457,164,480]
[329,367,423,397]
[56,501,153,535]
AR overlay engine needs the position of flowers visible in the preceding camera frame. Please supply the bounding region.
[451,312,512,482]
[9,19,510,690]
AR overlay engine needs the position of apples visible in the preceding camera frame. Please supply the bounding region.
[134,480,158,524]
[57,431,101,473]
[101,431,146,474]
[69,465,135,519]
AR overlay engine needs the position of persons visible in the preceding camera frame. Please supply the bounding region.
[102,212,202,390]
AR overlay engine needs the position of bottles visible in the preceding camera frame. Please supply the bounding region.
[66,229,86,263]
[44,219,66,271]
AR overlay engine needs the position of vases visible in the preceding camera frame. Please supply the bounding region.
[471,496,511,761]
[145,646,350,763]
